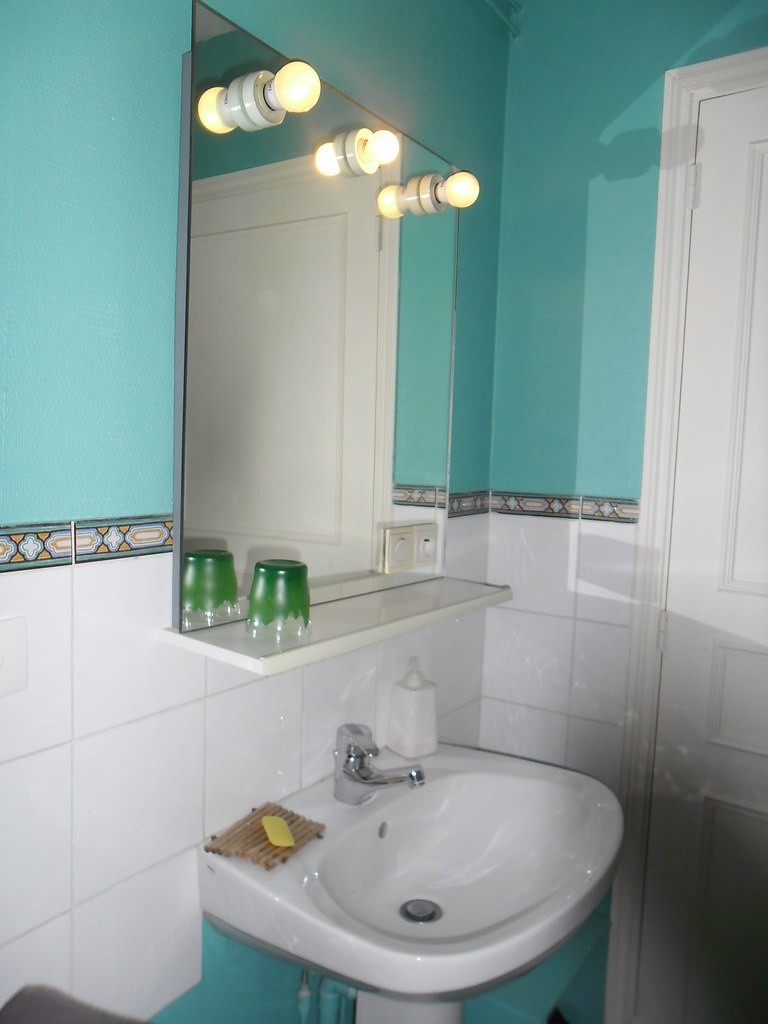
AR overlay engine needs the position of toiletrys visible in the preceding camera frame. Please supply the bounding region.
[386,655,439,760]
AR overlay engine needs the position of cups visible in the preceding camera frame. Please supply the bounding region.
[181,550,241,632]
[246,559,311,641]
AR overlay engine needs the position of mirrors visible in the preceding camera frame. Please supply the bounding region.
[177,0,459,631]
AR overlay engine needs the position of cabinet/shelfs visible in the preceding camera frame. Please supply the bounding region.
[199,883,612,1024]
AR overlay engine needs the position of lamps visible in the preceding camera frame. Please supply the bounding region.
[376,176,426,219]
[198,75,262,134]
[345,128,400,175]
[315,132,356,178]
[242,62,321,128]
[419,172,479,214]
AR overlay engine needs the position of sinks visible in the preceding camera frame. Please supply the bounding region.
[314,766,626,1003]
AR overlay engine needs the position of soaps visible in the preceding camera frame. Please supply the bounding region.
[261,815,295,847]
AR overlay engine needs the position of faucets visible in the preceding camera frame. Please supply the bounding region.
[332,722,426,806]
